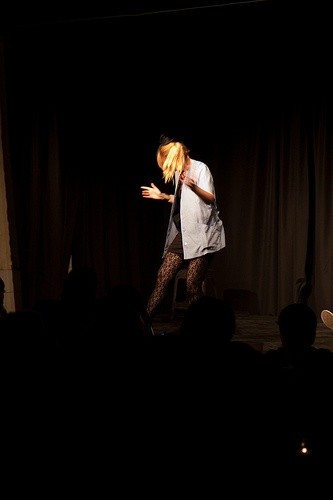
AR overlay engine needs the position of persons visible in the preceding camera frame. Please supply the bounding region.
[0,277,333,500]
[140,134,226,329]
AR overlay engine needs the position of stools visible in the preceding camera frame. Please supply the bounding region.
[171,269,219,315]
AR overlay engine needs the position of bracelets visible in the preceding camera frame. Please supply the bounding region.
[162,193,165,200]
